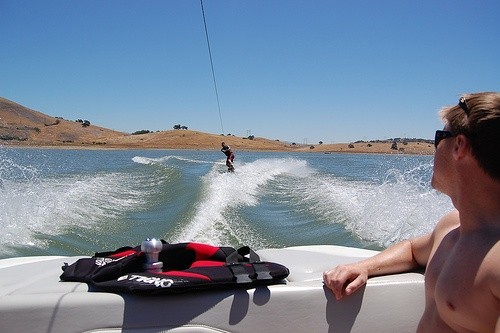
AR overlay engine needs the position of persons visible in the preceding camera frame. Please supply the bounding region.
[323,91,500,333]
[220,142,235,170]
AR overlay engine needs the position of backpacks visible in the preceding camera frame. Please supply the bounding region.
[60,239,290,296]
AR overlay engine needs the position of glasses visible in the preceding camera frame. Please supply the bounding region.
[434,130,464,148]
